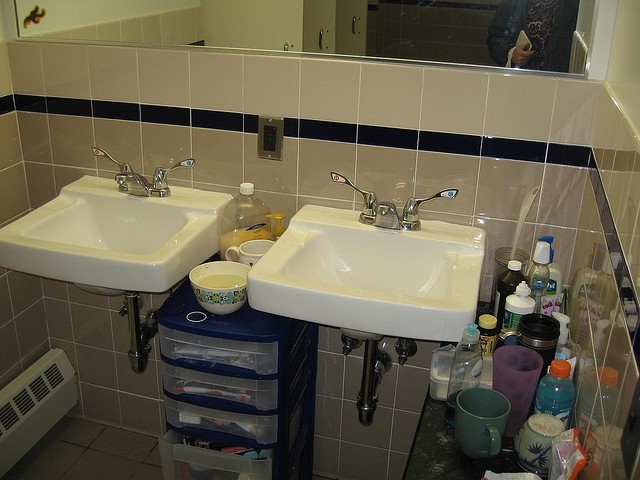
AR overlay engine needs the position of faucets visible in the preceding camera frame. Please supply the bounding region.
[114,172,152,198]
[375,202,402,233]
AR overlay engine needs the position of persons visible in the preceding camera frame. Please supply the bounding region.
[486,0,584,75]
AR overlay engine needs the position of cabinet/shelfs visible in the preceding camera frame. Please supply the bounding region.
[154,252,319,480]
[202,2,370,57]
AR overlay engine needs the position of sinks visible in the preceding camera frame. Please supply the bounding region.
[246,202,485,339]
[1,174,236,297]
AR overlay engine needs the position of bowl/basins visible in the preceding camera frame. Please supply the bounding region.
[189,261,250,315]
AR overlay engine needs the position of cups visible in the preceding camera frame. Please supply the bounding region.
[457,388,511,461]
[493,345,543,438]
[225,240,276,267]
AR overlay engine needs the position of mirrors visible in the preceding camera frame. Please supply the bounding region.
[11,1,621,81]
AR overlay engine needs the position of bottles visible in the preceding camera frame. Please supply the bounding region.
[219,182,271,263]
[537,358,574,429]
[495,259,522,321]
